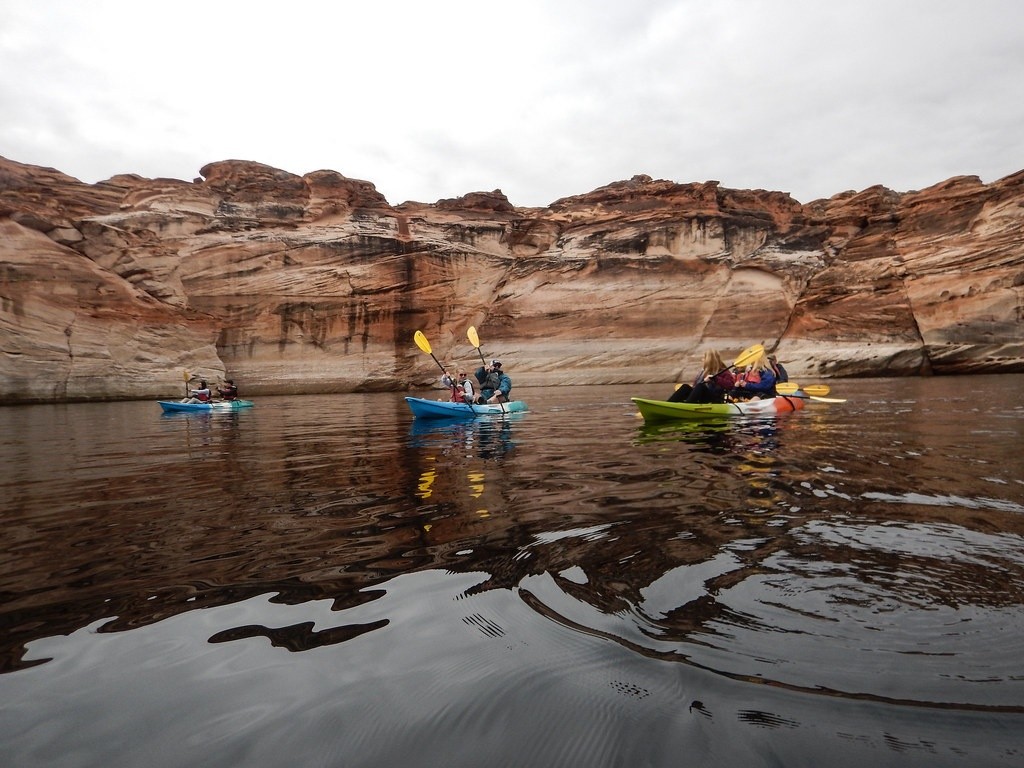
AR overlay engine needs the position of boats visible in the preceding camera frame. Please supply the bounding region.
[634,394,806,418]
[406,395,526,417]
[157,400,251,414]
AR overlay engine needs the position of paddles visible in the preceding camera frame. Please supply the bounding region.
[215,386,222,395]
[797,385,830,396]
[414,330,479,416]
[775,382,799,395]
[784,396,847,403]
[692,344,764,389]
[467,325,506,414]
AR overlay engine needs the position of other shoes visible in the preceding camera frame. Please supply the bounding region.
[478,397,487,405]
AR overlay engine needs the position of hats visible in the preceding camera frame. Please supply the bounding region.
[490,359,502,367]
[224,379,233,385]
[767,354,777,361]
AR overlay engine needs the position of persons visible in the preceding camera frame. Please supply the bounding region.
[667,349,788,404]
[170,381,237,404]
[421,359,511,403]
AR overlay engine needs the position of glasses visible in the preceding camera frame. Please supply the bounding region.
[459,373,467,376]
[491,364,500,368]
